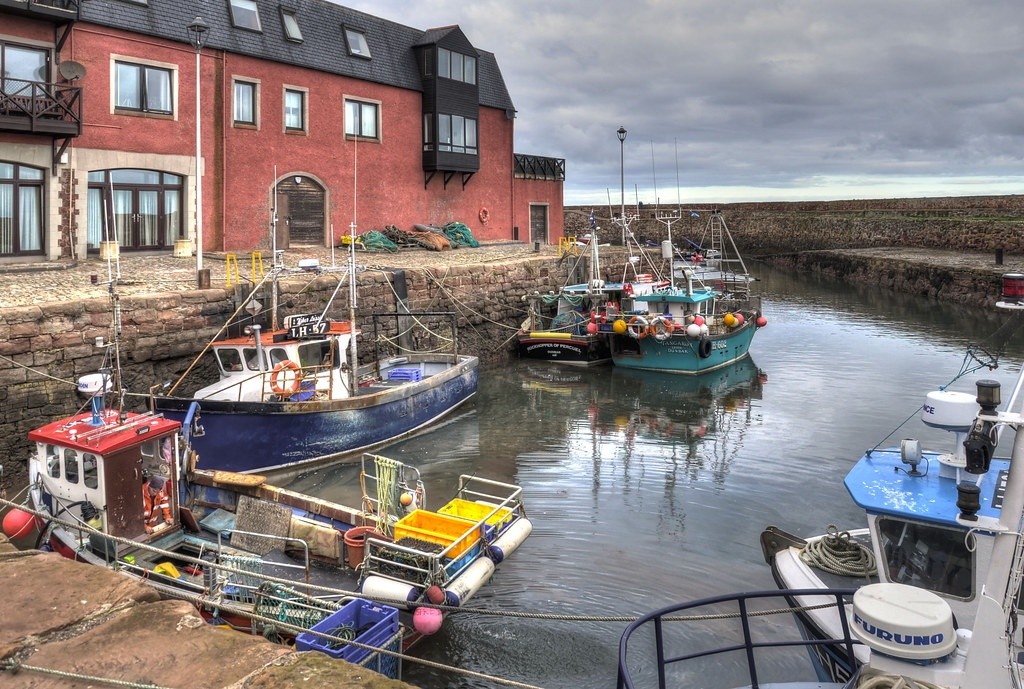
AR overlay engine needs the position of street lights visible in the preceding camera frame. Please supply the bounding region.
[185,16,215,274]
[615,124,628,248]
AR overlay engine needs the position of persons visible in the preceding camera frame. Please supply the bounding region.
[142,477,172,533]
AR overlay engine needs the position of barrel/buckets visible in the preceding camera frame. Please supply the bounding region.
[344,526,381,569]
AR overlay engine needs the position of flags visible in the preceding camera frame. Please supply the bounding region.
[690,212,699,217]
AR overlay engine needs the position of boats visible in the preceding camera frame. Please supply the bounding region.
[616,270,1024,689]
[145,116,480,477]
[515,136,762,375]
[758,378,1024,683]
[24,172,534,658]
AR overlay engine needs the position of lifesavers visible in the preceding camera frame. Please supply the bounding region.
[634,274,652,283]
[699,338,712,358]
[270,360,300,397]
[652,282,670,292]
[479,207,489,222]
[628,316,649,339]
[650,317,671,340]
